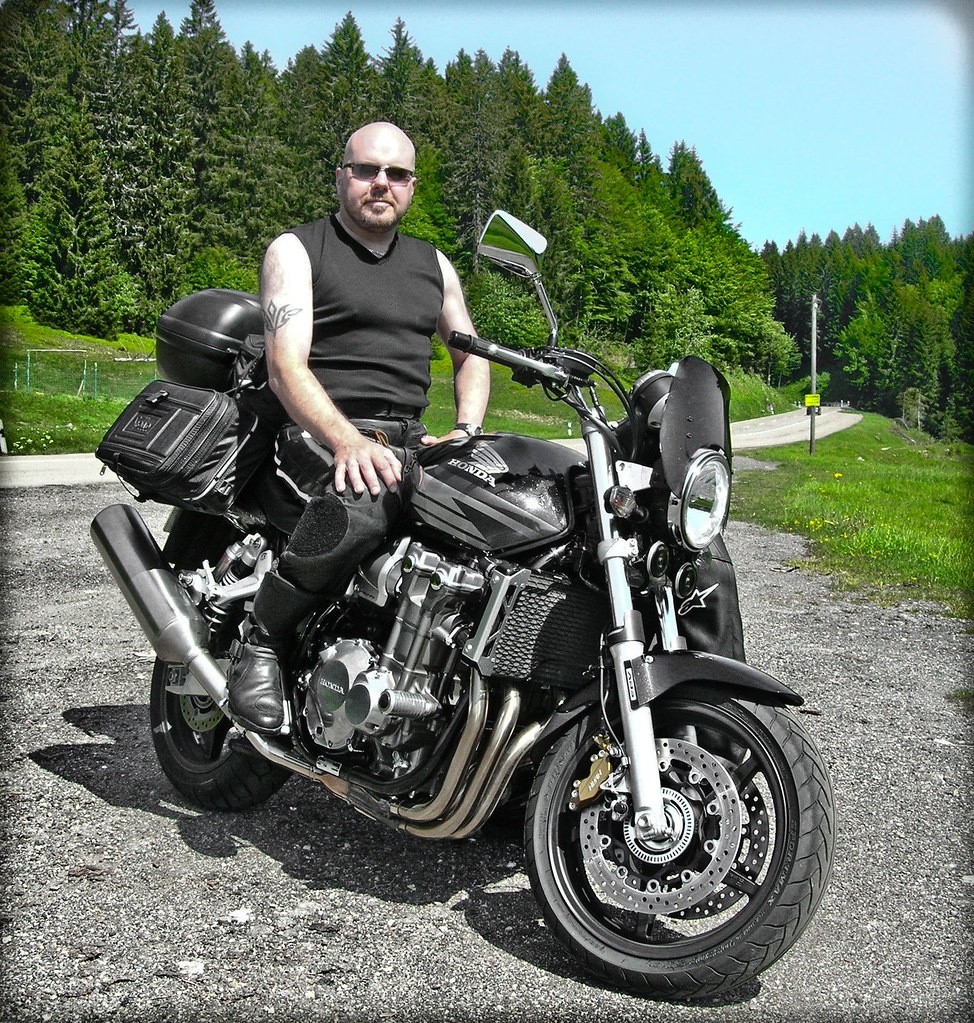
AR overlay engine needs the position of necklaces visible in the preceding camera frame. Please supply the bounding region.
[367,248,387,256]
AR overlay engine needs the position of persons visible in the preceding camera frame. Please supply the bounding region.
[225,124,490,734]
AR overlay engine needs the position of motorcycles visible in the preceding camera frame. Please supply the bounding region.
[87,203,838,993]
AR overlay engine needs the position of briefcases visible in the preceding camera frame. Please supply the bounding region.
[95,379,278,516]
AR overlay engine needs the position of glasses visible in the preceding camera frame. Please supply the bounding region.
[342,163,413,185]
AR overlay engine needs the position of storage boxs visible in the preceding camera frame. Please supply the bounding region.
[155,289,264,394]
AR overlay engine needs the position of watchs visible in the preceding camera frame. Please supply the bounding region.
[454,423,483,436]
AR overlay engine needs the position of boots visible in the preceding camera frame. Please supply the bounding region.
[228,557,322,737]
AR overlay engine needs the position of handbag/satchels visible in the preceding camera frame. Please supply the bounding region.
[225,333,289,427]
